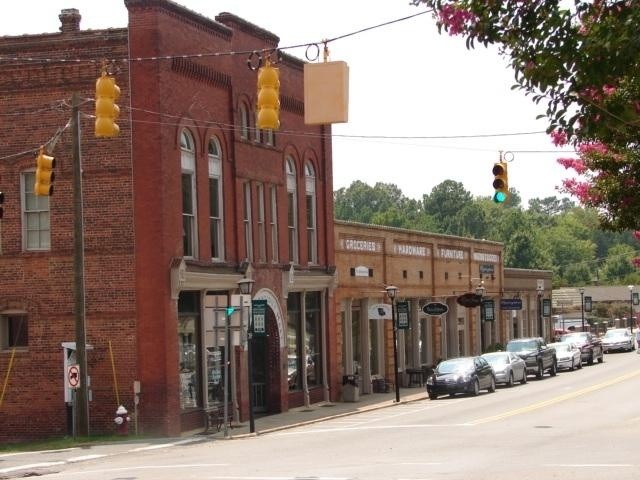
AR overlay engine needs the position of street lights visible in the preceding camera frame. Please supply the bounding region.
[537,290,545,337]
[578,287,585,332]
[475,284,485,353]
[237,257,256,434]
[628,284,635,330]
[384,283,400,404]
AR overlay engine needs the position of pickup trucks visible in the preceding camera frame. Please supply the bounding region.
[559,332,604,365]
[506,336,558,380]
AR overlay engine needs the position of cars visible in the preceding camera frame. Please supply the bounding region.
[554,328,571,335]
[287,354,314,389]
[601,329,635,353]
[427,355,497,400]
[547,342,582,371]
[480,351,528,387]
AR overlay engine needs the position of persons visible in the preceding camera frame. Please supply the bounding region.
[634,323,640,354]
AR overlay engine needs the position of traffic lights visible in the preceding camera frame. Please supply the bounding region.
[0,192,4,217]
[492,161,509,205]
[114,85,121,138]
[35,146,57,197]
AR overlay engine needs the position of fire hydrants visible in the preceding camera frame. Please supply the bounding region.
[115,405,131,439]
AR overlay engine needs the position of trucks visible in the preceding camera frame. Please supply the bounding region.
[552,314,588,332]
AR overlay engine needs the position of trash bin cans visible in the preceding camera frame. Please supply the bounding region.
[343,375,359,402]
